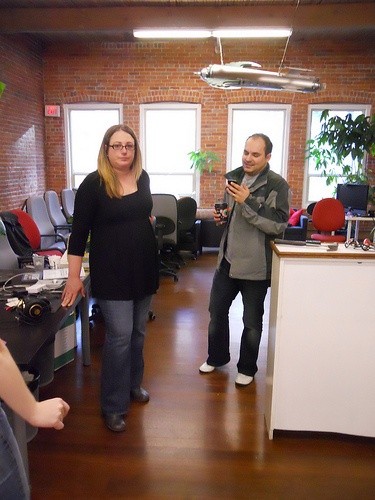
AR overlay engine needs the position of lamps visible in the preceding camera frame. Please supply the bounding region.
[130,24,294,39]
[191,37,327,97]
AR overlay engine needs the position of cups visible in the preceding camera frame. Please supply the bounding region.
[32,255,45,273]
[48,255,61,270]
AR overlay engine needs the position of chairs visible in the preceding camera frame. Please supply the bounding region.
[21,194,68,254]
[10,207,63,258]
[152,215,182,282]
[178,198,204,261]
[0,217,50,271]
[61,188,75,219]
[45,190,73,244]
[310,197,347,243]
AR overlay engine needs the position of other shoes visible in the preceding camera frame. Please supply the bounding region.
[199,361,215,372]
[235,372,253,385]
[132,388,150,402]
[104,413,127,431]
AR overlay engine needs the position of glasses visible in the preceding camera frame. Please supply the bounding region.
[108,144,136,150]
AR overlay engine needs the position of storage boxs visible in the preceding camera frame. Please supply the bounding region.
[54,308,76,371]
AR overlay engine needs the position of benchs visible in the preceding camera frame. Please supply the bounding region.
[283,215,309,241]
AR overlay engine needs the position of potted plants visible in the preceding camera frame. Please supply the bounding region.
[303,108,375,216]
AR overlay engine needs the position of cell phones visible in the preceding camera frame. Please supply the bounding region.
[227,180,237,195]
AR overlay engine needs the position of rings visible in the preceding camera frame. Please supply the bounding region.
[232,194,235,197]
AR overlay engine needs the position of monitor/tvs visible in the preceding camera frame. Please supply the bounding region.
[336,183,368,210]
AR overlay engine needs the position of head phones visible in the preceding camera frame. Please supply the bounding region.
[15,296,52,326]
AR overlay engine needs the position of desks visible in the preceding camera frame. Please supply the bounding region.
[345,215,375,245]
[0,271,91,488]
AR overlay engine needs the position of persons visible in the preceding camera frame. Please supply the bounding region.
[0,338,70,500]
[61,124,160,431]
[199,134,290,385]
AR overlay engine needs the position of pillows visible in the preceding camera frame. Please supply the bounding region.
[290,207,296,217]
[288,210,304,226]
